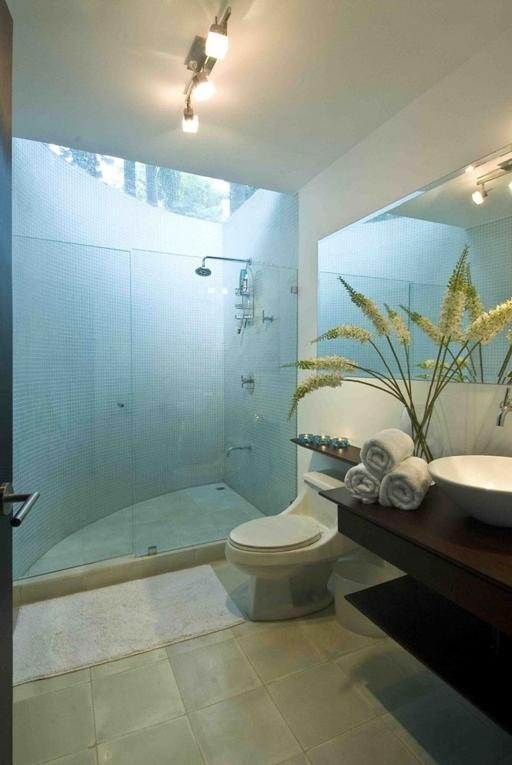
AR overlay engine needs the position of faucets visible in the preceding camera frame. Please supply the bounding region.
[495,386,512,428]
[226,444,252,457]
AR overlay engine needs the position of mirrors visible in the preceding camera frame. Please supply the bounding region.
[318,143,512,384]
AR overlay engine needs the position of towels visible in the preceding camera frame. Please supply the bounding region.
[360,427,415,483]
[378,454,432,512]
[344,462,381,506]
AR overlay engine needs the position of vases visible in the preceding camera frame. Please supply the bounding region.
[399,405,443,460]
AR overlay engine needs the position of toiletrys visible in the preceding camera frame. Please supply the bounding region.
[239,269,248,296]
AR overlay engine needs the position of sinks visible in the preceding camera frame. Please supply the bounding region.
[426,454,512,527]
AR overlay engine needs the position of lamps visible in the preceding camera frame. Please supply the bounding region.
[471,165,512,205]
[182,7,232,133]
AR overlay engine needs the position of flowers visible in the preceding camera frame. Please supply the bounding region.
[282,244,512,467]
[416,262,512,384]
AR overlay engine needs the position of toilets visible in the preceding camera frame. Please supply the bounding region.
[225,434,359,621]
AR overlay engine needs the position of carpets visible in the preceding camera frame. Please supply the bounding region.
[12,562,245,687]
